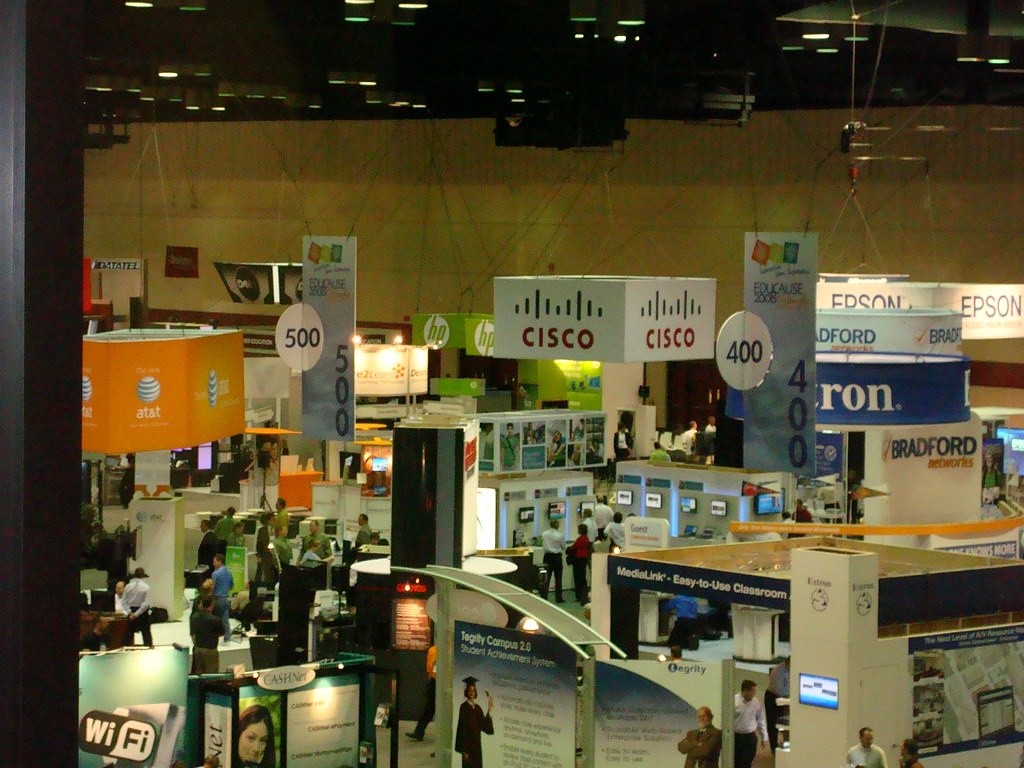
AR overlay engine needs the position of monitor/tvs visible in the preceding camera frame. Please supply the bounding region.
[617,490,632,505]
[754,492,780,515]
[580,501,595,517]
[644,493,662,508]
[549,501,567,519]
[680,497,697,514]
[710,500,728,517]
[518,507,535,523]
[799,674,839,710]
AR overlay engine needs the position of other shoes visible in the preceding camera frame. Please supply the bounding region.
[405,732,423,741]
[556,599,566,603]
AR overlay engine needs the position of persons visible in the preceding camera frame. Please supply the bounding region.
[118,453,136,508]
[405,643,437,741]
[897,739,925,768]
[649,441,671,463]
[841,727,888,768]
[195,755,219,768]
[611,422,635,482]
[191,597,227,675]
[500,418,604,470]
[781,498,814,539]
[665,593,699,651]
[734,679,768,768]
[764,654,790,757]
[455,676,495,768]
[239,705,276,768]
[279,439,289,455]
[539,494,636,606]
[111,496,390,648]
[847,467,861,524]
[81,592,107,652]
[682,415,717,466]
[678,707,722,768]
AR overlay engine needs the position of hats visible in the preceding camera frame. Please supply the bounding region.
[132,568,149,578]
[463,676,479,685]
